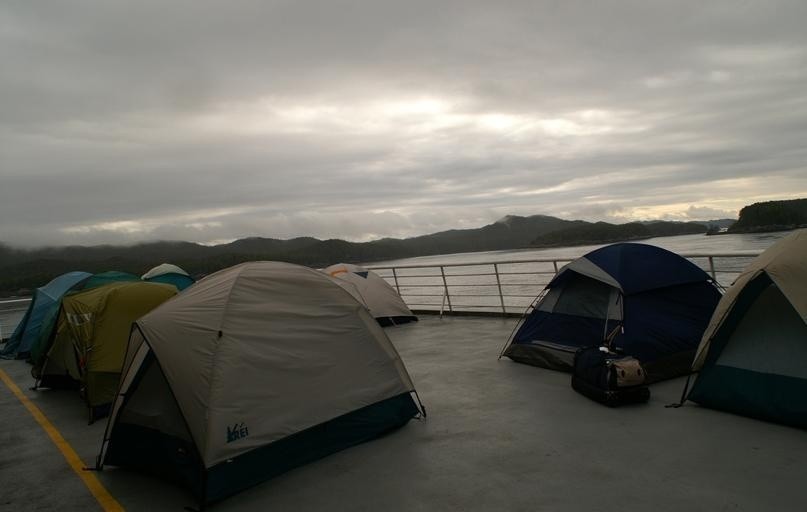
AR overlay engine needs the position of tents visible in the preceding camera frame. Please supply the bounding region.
[26,270,140,373]
[319,263,417,328]
[140,262,195,292]
[497,242,725,387]
[662,226,806,429]
[31,280,180,425]
[94,258,427,508]
[0,270,97,360]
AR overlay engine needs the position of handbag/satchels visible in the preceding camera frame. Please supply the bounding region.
[570,340,650,407]
